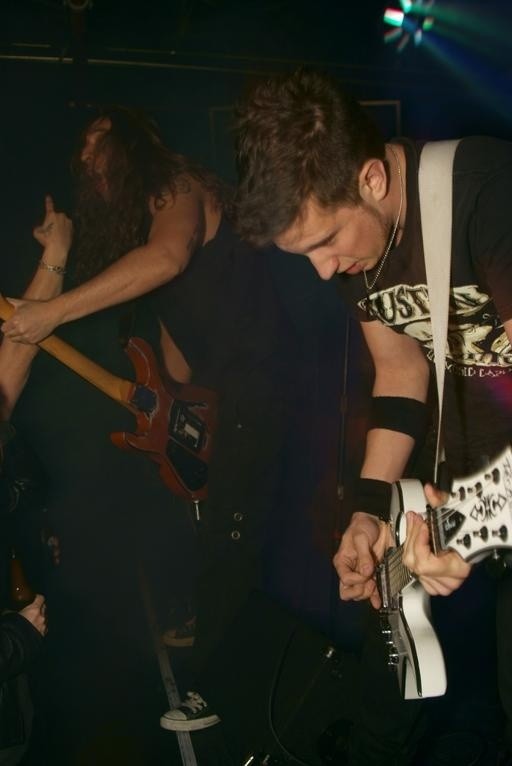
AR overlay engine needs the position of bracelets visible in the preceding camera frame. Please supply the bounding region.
[36,260,66,278]
[347,472,393,525]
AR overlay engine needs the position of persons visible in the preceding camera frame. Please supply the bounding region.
[0,591,50,766]
[0,194,77,426]
[0,114,286,738]
[212,63,511,766]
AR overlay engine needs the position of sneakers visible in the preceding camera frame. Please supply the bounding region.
[163,617,196,646]
[160,689,220,732]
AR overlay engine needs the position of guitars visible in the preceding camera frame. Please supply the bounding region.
[374,443,512,700]
[0,295,216,501]
